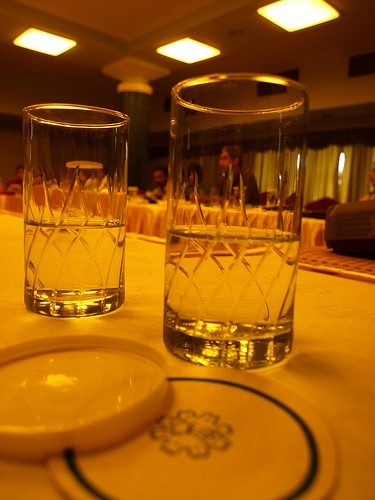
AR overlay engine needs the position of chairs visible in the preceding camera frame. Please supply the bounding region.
[304,197,340,214]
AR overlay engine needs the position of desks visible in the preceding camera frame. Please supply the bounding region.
[0,208,375,500]
[5,186,326,248]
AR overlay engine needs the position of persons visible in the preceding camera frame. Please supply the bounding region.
[0,146,374,206]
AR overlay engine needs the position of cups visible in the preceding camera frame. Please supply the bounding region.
[23,104,128,316]
[164,74,307,370]
